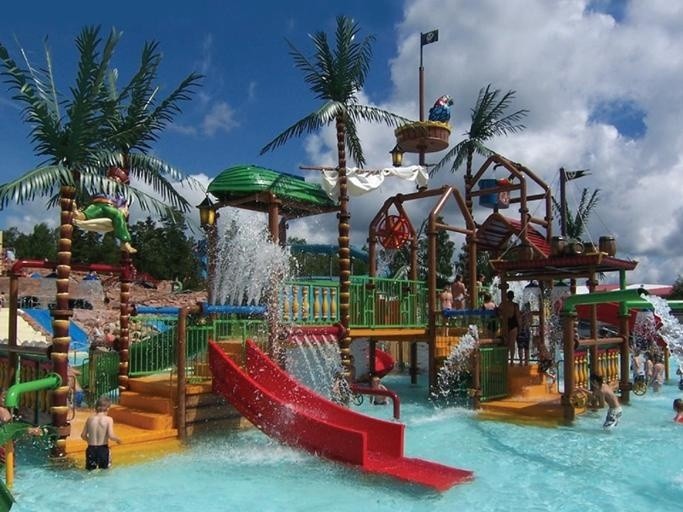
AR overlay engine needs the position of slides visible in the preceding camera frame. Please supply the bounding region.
[374,347,395,377]
[207,340,474,491]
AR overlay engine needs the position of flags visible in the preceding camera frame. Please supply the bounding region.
[422,29,438,46]
[566,169,592,181]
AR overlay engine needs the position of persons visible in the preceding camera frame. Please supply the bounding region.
[81,396,122,471]
[439,271,541,366]
[579,373,623,427]
[631,349,665,392]
[92,318,103,337]
[104,326,115,343]
[672,398,683,424]
[71,167,137,253]
